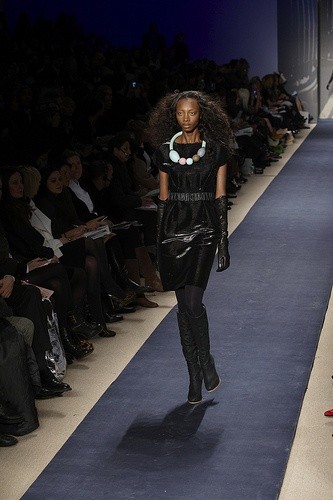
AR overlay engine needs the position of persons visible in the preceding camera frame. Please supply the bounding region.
[147,90,234,404]
[0,58,314,447]
[326,71,333,91]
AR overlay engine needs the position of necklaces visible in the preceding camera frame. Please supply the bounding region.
[169,130,206,166]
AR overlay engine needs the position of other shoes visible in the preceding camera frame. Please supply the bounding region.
[115,304,135,313]
[72,315,102,339]
[136,294,159,308]
[106,288,136,308]
[40,368,72,391]
[62,329,94,360]
[33,386,63,400]
[99,323,116,338]
[104,312,123,323]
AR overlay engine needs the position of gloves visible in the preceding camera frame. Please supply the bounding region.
[155,199,168,272]
[216,195,231,272]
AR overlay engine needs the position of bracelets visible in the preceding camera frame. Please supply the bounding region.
[63,232,71,241]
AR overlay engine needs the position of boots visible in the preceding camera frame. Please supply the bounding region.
[176,304,221,405]
